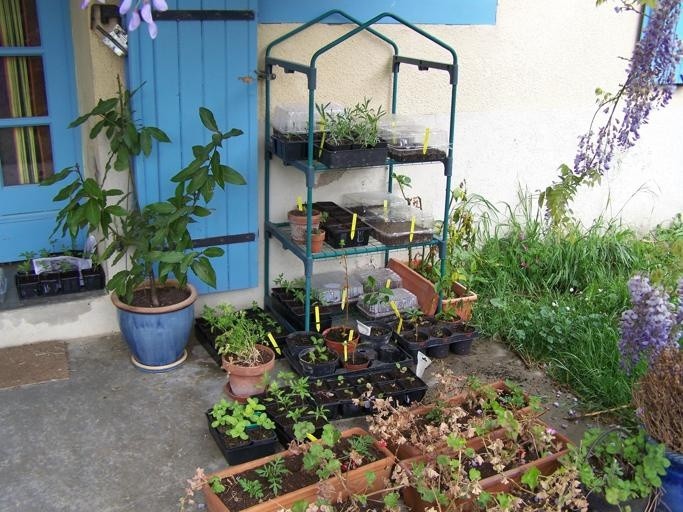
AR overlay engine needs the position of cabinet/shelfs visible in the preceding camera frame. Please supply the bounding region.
[262,9,459,350]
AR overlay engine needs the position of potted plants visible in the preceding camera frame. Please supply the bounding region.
[13,249,105,302]
[36,74,248,375]
